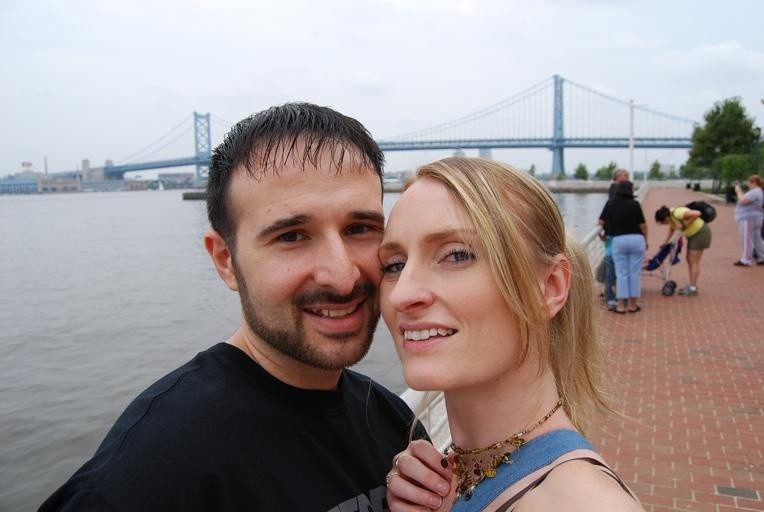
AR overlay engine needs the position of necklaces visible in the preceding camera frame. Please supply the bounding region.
[444,396,564,501]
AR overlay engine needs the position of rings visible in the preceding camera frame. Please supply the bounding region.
[393,453,408,468]
[384,471,398,487]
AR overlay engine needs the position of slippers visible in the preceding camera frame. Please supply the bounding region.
[617,306,639,313]
[734,259,764,266]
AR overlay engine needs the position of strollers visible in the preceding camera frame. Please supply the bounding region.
[644,236,683,295]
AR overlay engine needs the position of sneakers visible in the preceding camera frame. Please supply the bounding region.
[679,284,698,296]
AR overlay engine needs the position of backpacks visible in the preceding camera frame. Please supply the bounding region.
[672,201,716,222]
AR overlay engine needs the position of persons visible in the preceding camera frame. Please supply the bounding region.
[598,181,647,314]
[654,204,711,296]
[36,102,433,511]
[733,175,764,266]
[607,169,630,201]
[600,229,617,307]
[378,157,644,512]
